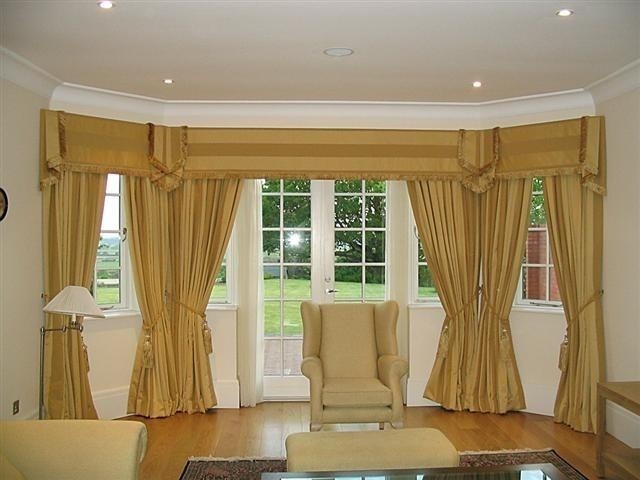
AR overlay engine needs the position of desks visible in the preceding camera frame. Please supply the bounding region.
[593,381,640,480]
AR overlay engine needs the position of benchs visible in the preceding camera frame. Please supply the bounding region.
[284,427,460,471]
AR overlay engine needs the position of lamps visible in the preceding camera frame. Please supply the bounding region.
[37,285,106,421]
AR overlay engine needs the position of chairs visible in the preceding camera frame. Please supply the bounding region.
[299,297,411,429]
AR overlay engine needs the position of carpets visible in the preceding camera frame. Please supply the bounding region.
[178,446,589,479]
